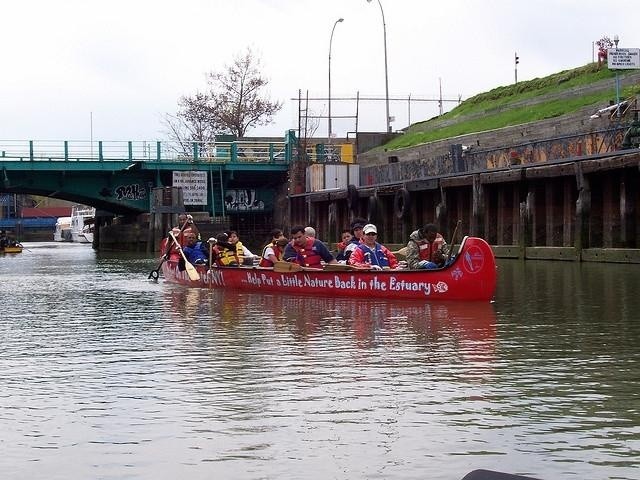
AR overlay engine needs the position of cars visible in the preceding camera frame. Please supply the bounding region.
[272,146,313,163]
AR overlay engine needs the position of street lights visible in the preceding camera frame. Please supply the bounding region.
[327,17,344,139]
[368,0,390,133]
[614,33,622,119]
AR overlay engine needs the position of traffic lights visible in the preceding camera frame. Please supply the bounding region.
[515,55,519,65]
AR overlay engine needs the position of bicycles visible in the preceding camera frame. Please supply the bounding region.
[324,148,342,164]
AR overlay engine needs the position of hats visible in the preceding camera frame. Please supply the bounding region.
[363,223,378,236]
[214,231,231,241]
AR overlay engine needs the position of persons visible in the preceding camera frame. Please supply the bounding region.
[159,212,457,272]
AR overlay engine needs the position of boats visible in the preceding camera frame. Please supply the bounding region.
[53,204,97,244]
[0,244,23,253]
[161,234,498,303]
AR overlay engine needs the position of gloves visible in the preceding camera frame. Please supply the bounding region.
[394,265,407,270]
[420,259,437,269]
[369,265,383,271]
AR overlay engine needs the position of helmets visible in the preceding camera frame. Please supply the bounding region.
[186,214,194,224]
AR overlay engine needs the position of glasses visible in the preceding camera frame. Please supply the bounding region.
[365,233,377,238]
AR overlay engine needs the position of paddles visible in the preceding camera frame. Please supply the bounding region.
[274,262,323,272]
[169,231,200,280]
[323,264,373,272]
[390,247,407,255]
[148,217,189,281]
